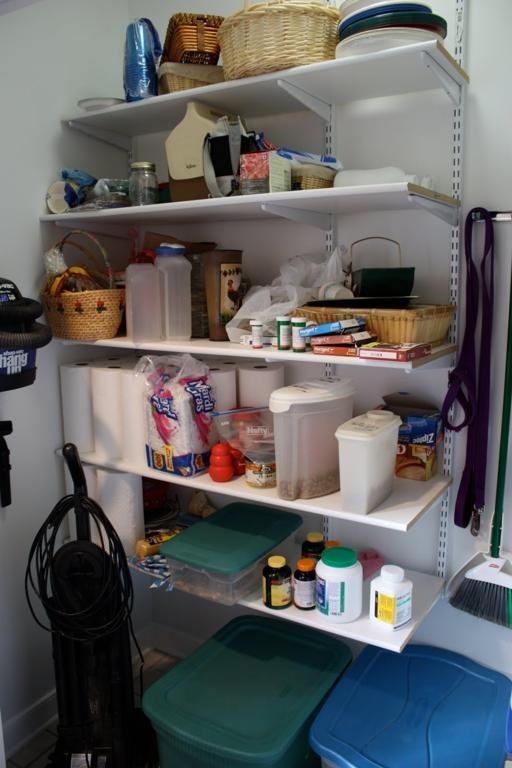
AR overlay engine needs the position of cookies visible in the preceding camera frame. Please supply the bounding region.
[396,457,427,479]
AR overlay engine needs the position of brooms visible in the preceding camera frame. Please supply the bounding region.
[448,264,512,629]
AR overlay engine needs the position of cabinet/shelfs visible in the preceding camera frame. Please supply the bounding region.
[39,39,469,653]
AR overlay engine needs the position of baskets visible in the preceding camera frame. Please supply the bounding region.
[242,165,336,194]
[219,0,340,84]
[276,298,455,349]
[158,11,225,64]
[153,62,223,96]
[42,228,127,343]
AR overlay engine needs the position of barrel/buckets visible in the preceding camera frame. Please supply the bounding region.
[125,255,166,342]
[153,241,194,343]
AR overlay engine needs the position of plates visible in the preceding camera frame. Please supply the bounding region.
[335,0,447,60]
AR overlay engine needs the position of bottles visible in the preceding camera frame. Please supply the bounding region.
[292,558,317,610]
[124,245,193,344]
[369,565,413,632]
[128,161,161,206]
[276,316,291,350]
[301,532,326,569]
[250,320,264,349]
[262,555,292,609]
[315,547,363,624]
[291,317,307,352]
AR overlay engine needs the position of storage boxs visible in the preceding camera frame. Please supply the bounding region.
[142,613,354,768]
[159,501,302,608]
[308,643,511,768]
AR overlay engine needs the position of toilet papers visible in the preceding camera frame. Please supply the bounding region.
[96,469,146,557]
[57,354,286,475]
[62,459,100,545]
